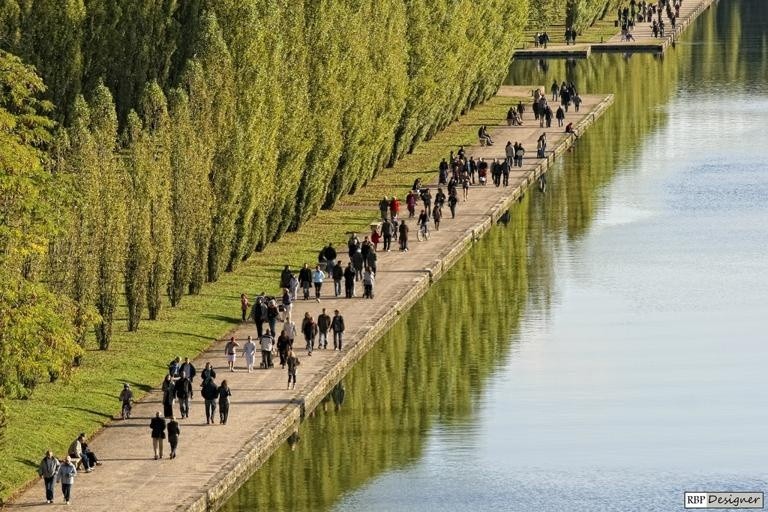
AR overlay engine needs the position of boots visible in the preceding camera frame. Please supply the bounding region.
[286,382,296,390]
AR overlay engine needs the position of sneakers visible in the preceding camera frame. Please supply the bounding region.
[383,248,408,253]
[82,461,102,473]
[153,452,177,461]
[44,498,70,506]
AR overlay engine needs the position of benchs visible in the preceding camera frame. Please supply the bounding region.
[317,258,336,275]
[507,115,519,125]
[370,218,385,236]
[480,134,493,146]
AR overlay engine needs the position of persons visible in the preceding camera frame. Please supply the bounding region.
[534,0,683,49]
